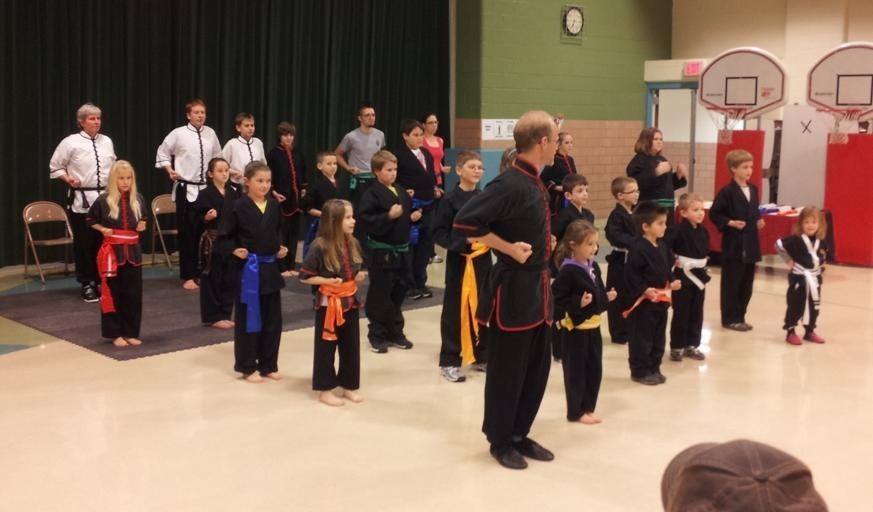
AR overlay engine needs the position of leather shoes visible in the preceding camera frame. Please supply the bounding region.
[489,442,527,469]
[516,438,553,461]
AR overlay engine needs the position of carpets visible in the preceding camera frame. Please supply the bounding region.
[0,261,445,361]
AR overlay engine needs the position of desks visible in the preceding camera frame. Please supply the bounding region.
[675,197,826,256]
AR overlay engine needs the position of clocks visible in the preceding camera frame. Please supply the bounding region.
[559,4,585,44]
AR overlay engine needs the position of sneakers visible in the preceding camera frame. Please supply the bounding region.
[786,333,800,345]
[407,289,421,300]
[722,323,747,331]
[80,286,99,303]
[418,287,432,297]
[431,255,442,263]
[440,366,464,382]
[471,362,487,372]
[387,337,412,350]
[370,342,387,353]
[631,346,704,385]
[803,332,824,343]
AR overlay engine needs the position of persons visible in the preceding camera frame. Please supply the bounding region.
[772,205,829,345]
[155,97,766,424]
[450,110,561,469]
[48,102,118,303]
[86,158,149,348]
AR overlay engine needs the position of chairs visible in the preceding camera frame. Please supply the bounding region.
[22,201,74,283]
[150,194,178,270]
